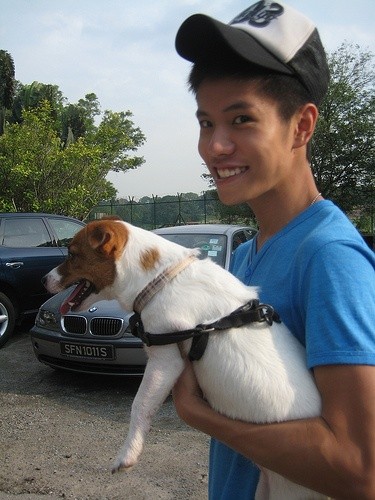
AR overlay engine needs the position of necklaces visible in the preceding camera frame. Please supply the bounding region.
[309,192,322,206]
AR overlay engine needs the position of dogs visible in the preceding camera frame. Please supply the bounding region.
[42,213,336,500]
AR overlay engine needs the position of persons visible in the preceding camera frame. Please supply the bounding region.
[173,0,375,500]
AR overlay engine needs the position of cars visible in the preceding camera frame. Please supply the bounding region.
[28,224,259,379]
[0,212,90,347]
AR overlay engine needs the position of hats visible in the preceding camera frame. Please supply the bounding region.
[175,0,330,105]
